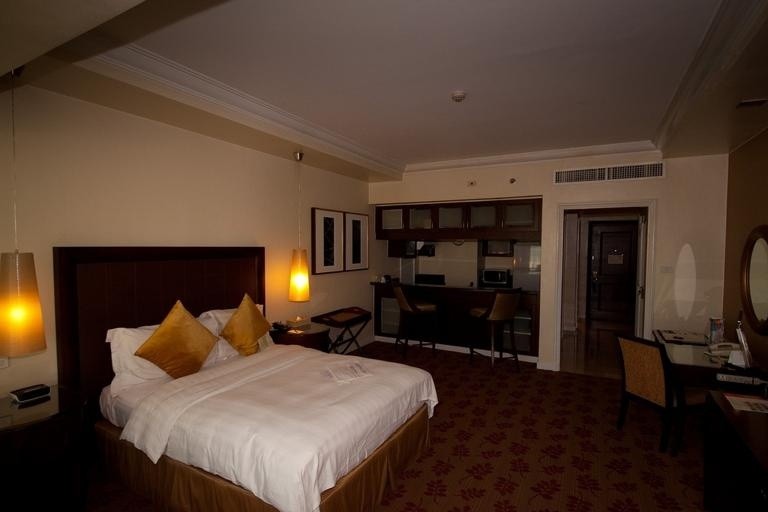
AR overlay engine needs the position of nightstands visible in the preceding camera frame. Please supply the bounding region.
[0,386,87,511]
[278,322,330,352]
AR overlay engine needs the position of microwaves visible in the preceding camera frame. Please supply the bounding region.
[481,269,510,286]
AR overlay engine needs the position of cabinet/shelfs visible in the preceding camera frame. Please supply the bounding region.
[375,199,541,241]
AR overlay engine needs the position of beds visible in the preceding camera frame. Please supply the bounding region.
[51,247,441,512]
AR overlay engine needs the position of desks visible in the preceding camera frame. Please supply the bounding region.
[653,329,760,404]
[312,306,371,354]
[704,390,767,512]
[370,280,539,356]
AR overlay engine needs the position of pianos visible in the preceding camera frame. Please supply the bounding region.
[311,306,372,329]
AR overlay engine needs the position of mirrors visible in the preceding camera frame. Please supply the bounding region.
[740,226,768,334]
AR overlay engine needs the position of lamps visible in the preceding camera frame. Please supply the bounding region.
[289,152,311,303]
[0,64,47,357]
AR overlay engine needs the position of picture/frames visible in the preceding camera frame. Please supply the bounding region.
[311,207,344,274]
[345,212,369,271]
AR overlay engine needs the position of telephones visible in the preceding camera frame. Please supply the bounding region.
[272,321,290,332]
[709,343,740,356]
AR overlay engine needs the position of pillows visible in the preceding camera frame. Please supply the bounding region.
[219,292,271,357]
[106,325,168,394]
[197,304,276,359]
[134,299,220,378]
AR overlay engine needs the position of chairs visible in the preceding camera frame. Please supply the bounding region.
[468,287,522,368]
[392,285,437,353]
[615,334,707,452]
